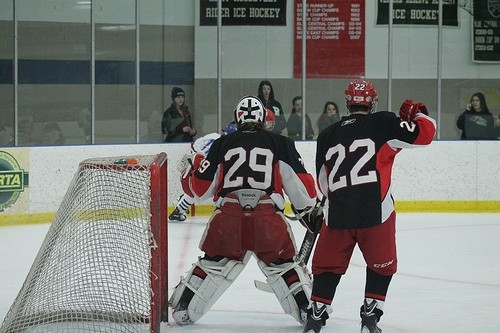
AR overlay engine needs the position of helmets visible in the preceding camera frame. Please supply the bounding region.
[233,95,266,129]
[344,79,378,114]
[265,108,276,123]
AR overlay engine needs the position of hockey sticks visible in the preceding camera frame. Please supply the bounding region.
[253,196,327,292]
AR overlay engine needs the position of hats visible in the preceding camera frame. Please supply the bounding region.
[171,87,185,100]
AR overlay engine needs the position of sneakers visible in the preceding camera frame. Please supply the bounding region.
[172,308,193,325]
[302,301,329,333]
[169,207,186,221]
[300,309,310,324]
[360,299,383,333]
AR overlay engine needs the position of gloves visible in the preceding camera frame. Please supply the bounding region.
[194,154,205,171]
[399,99,429,123]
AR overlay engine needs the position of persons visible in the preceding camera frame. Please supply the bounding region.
[457,92,500,140]
[167,95,329,327]
[162,81,341,221]
[299,81,437,333]
